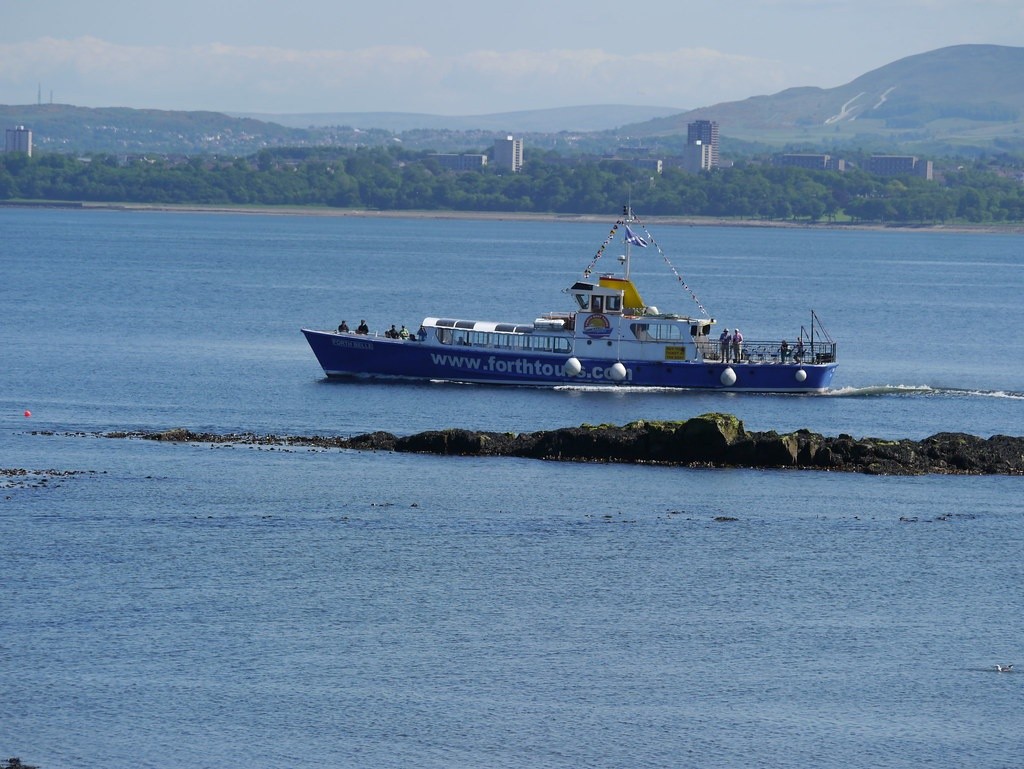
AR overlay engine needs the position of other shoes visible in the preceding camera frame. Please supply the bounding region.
[737,361,740,363]
[727,361,728,363]
[721,361,723,363]
[733,360,737,363]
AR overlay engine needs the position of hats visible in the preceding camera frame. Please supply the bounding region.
[735,329,739,332]
[723,328,729,332]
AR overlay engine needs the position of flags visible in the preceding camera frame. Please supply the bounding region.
[625,225,648,248]
[584,225,618,279]
[643,226,704,314]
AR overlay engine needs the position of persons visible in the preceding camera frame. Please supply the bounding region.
[719,328,732,363]
[399,325,409,340]
[781,340,788,362]
[338,320,349,333]
[389,325,400,338]
[794,337,804,364]
[417,325,427,339]
[733,329,743,363]
[357,320,368,335]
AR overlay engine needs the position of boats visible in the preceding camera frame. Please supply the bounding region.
[299,201,839,394]
